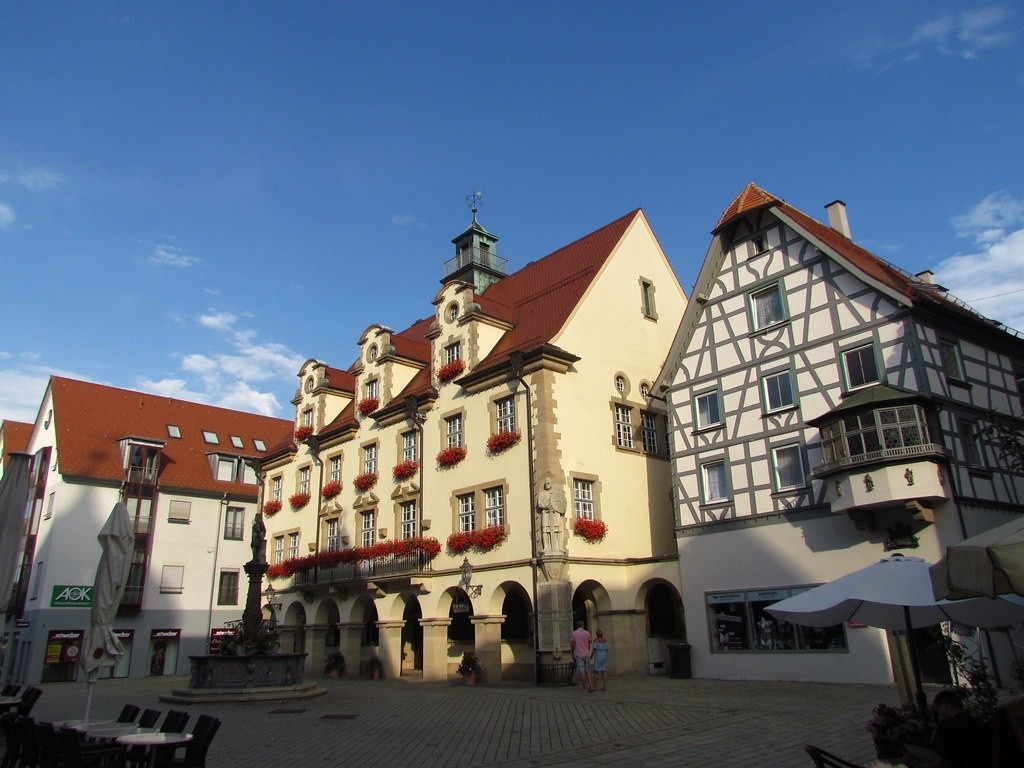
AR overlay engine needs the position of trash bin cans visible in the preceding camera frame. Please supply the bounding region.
[667,642,692,679]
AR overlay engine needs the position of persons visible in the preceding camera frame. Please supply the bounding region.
[589,629,608,692]
[251,514,266,562]
[570,620,593,692]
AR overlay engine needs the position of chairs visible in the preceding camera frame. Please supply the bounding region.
[0,683,54,768]
[157,714,222,768]
[128,708,161,760]
[110,704,140,743]
[131,710,190,761]
[55,727,126,768]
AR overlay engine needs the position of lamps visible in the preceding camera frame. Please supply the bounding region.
[460,556,482,596]
[264,584,282,611]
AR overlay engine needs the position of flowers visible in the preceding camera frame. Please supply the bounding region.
[262,500,281,514]
[392,459,417,481]
[864,703,921,738]
[437,359,463,381]
[575,516,608,542]
[447,524,504,555]
[358,397,378,416]
[287,490,310,509]
[354,473,377,490]
[485,429,521,452]
[436,446,468,468]
[319,478,343,499]
[294,424,313,438]
[265,537,441,579]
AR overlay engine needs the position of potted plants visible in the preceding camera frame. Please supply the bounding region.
[322,652,346,679]
[367,655,383,680]
[457,652,483,685]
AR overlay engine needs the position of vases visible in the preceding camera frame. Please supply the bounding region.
[874,736,896,759]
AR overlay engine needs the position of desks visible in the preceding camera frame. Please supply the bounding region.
[52,720,193,768]
[0,695,22,704]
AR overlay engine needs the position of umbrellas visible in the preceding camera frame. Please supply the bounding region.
[79,503,136,718]
[762,553,1023,708]
[928,516,1024,602]
[0,451,33,640]
